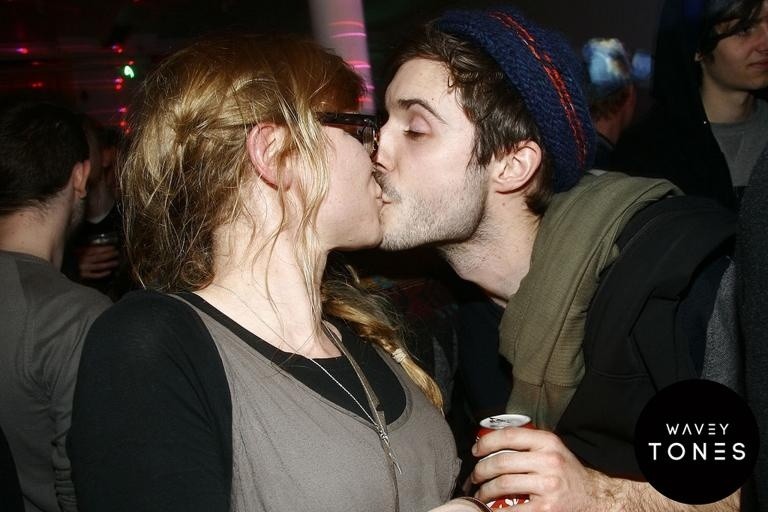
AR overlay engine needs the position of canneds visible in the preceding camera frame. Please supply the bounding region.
[475,413,537,511]
[91,231,119,245]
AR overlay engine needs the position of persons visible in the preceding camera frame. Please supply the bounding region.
[368,7,744,511]
[568,47,637,175]
[648,0,768,220]
[54,114,142,303]
[735,140,768,510]
[0,81,117,512]
[71,31,494,510]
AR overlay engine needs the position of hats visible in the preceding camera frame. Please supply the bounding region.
[431,7,597,192]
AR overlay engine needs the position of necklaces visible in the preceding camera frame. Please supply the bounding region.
[208,280,403,475]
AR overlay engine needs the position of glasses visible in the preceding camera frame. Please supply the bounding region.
[272,111,380,158]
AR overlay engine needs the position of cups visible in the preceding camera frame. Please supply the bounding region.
[91,232,117,248]
